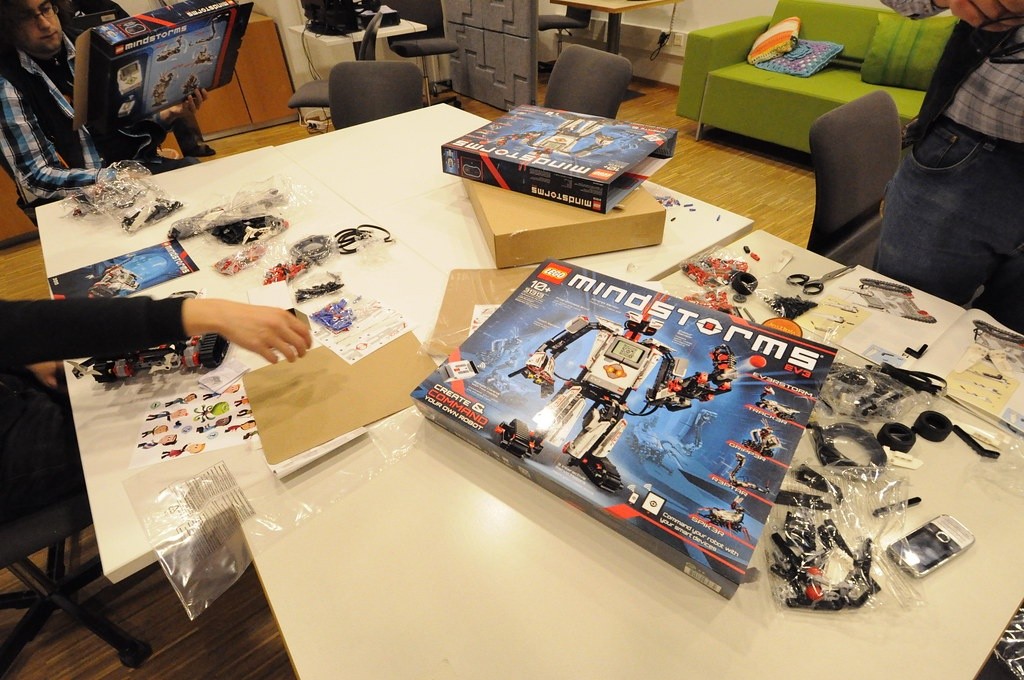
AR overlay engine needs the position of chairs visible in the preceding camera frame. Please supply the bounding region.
[0,493,154,680]
[382,0,462,109]
[807,88,903,262]
[539,4,592,72]
[327,61,425,130]
[287,5,391,108]
[542,44,633,122]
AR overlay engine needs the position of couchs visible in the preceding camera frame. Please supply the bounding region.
[676,0,958,158]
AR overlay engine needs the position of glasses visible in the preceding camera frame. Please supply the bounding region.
[15,4,59,25]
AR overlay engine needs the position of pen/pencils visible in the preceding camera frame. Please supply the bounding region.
[873,497,922,518]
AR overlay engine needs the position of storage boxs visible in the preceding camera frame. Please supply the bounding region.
[409,257,841,599]
[441,101,677,215]
[90,1,254,134]
[458,175,667,269]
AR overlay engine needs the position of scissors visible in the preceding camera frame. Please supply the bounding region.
[788,263,853,296]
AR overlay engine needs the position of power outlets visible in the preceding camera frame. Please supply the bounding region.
[659,32,670,45]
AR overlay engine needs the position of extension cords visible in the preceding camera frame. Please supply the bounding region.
[307,119,326,130]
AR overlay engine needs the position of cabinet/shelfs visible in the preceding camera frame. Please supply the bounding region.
[158,20,304,154]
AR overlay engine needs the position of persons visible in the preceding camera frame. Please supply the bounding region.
[870,0,1024,334]
[0,0,202,226]
[51,0,216,157]
[1,298,312,531]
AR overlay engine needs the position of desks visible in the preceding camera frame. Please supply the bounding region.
[287,18,427,62]
[227,228,1024,679]
[35,101,756,578]
[549,1,672,53]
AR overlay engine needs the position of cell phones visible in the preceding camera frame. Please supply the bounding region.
[886,510,976,580]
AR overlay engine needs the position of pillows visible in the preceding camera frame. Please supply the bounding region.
[860,9,957,89]
